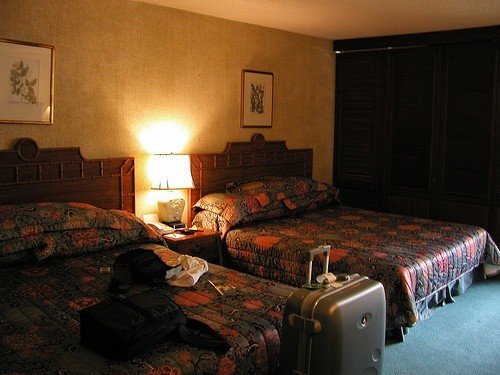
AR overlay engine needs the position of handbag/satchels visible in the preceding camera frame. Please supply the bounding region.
[78,286,232,361]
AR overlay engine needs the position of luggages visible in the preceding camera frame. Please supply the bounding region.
[278,243,386,375]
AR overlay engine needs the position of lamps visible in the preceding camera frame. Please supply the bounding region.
[150,153,196,225]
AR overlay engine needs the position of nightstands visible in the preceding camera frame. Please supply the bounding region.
[162,228,223,266]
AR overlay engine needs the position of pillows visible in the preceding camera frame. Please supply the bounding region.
[0,202,163,267]
[192,176,339,234]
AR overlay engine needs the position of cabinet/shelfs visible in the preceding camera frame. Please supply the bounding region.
[333,25,500,250]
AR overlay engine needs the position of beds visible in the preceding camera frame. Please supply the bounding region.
[187,133,500,342]
[0,137,301,375]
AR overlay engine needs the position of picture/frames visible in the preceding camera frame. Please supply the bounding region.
[240,69,274,128]
[0,38,54,125]
